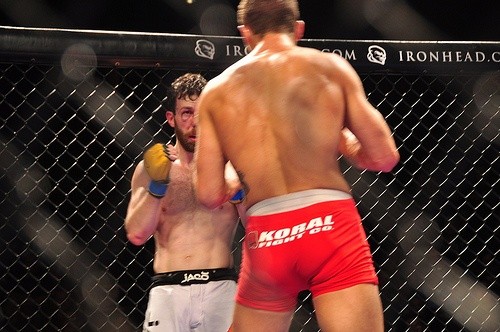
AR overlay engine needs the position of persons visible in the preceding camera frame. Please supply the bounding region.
[191,0,400,332]
[125,73,246,332]
